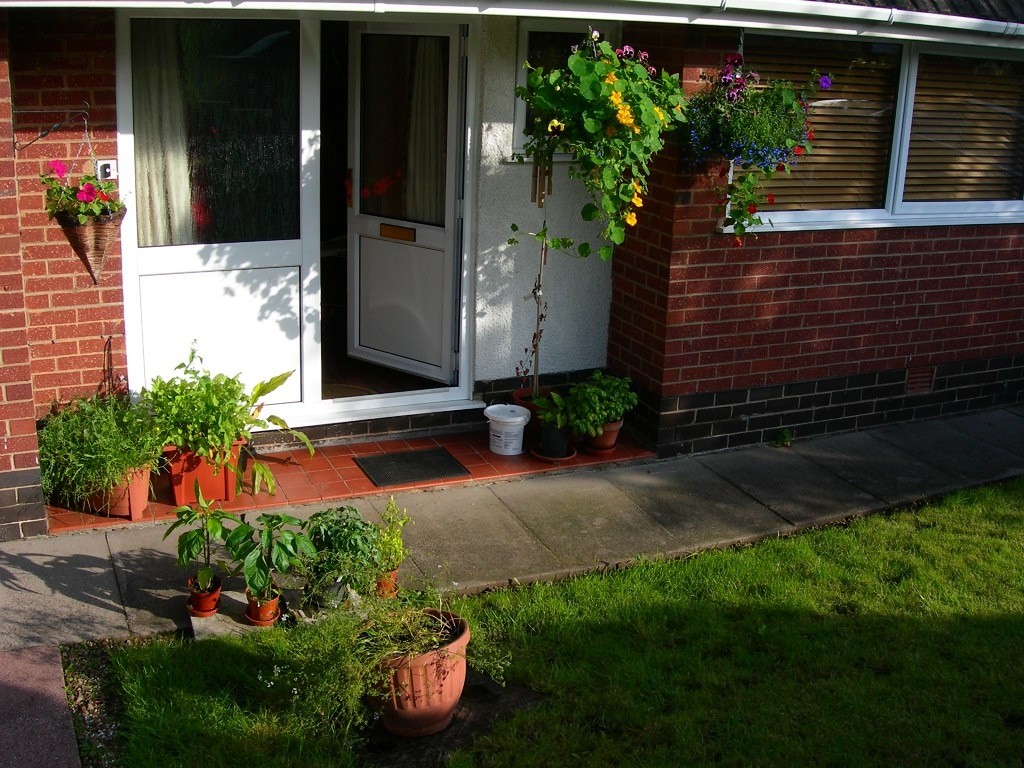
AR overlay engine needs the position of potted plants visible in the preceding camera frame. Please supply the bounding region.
[160,475,246,619]
[300,505,381,611]
[245,532,515,739]
[37,372,175,524]
[223,512,318,629]
[363,495,415,600]
[527,391,578,463]
[564,369,638,456]
[136,348,316,507]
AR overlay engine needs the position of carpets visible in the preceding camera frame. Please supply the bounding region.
[351,446,472,488]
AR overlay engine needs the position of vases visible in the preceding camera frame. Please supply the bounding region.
[512,386,561,430]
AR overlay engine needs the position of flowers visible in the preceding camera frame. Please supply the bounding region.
[505,29,692,262]
[40,159,126,225]
[677,52,839,240]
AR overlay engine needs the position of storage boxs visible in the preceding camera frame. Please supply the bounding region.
[482,403,531,456]
